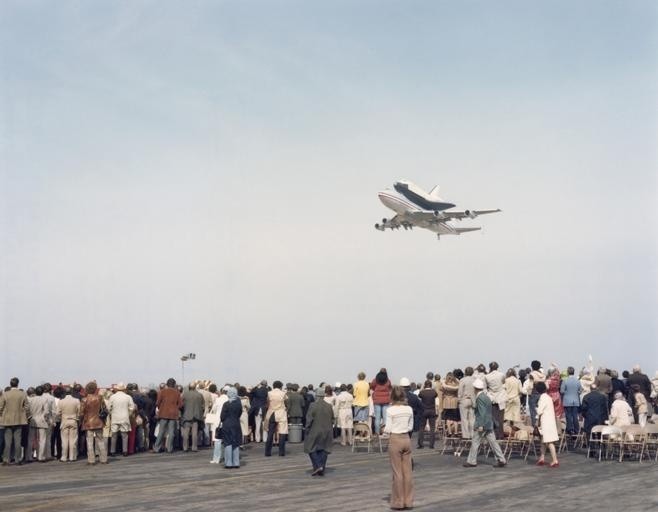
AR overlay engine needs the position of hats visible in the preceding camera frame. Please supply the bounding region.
[375,371,389,385]
[115,382,127,390]
[472,378,485,390]
[530,359,543,368]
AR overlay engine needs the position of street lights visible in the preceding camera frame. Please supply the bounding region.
[181,353,197,385]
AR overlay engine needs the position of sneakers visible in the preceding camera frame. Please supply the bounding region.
[223,465,241,469]
[209,459,220,464]
[123,452,129,457]
[390,505,414,511]
[462,463,477,467]
[340,439,353,446]
[311,466,326,476]
[110,452,117,457]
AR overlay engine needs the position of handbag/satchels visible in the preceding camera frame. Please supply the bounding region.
[204,408,220,424]
[215,427,225,439]
[97,401,110,420]
[532,425,545,442]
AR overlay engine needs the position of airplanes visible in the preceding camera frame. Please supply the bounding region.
[374,178,503,241]
[392,179,456,211]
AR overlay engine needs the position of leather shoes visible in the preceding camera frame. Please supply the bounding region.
[534,459,545,466]
[548,460,560,468]
[492,460,508,468]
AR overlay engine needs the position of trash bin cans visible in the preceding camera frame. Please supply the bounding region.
[288,423,302,442]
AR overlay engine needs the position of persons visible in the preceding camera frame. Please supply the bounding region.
[383,382,415,512]
[0,360,658,478]
